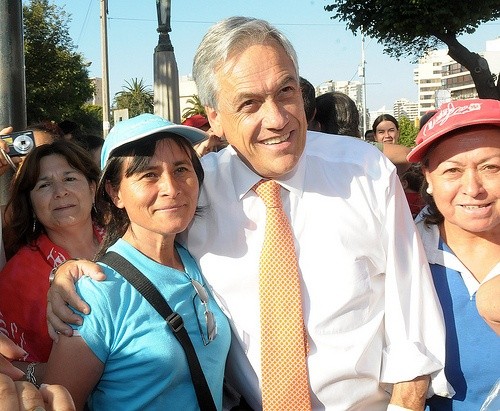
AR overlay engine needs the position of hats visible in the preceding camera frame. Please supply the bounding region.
[406,98,500,164]
[182,114,208,128]
[99,112,208,172]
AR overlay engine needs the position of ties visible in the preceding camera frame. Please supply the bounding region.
[251,180,313,411]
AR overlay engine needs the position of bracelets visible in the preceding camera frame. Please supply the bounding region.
[24,361,41,387]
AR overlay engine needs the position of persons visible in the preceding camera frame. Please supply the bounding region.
[0,15,500,411]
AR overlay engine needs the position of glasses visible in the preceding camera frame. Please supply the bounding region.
[188,276,219,345]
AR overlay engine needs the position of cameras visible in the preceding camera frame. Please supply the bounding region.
[0,130,36,157]
